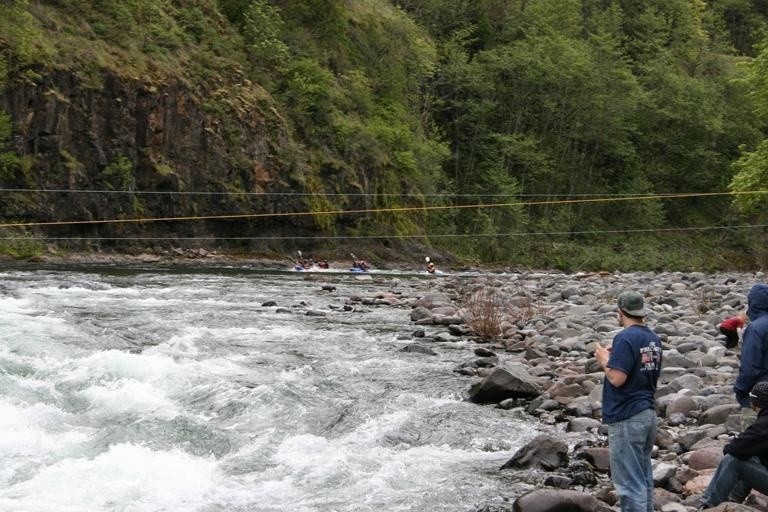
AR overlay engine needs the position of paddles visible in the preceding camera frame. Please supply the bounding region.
[424,256,430,268]
[297,250,309,269]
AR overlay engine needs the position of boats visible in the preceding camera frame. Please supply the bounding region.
[294,265,304,271]
[349,268,364,272]
[425,269,443,275]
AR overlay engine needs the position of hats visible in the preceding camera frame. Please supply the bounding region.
[748,381,768,409]
[617,290,648,317]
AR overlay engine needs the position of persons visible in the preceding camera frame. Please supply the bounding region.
[353,260,368,271]
[696,381,768,511]
[733,284,768,432]
[719,314,747,349]
[426,263,436,274]
[318,258,328,269]
[593,290,662,512]
[298,258,313,270]
